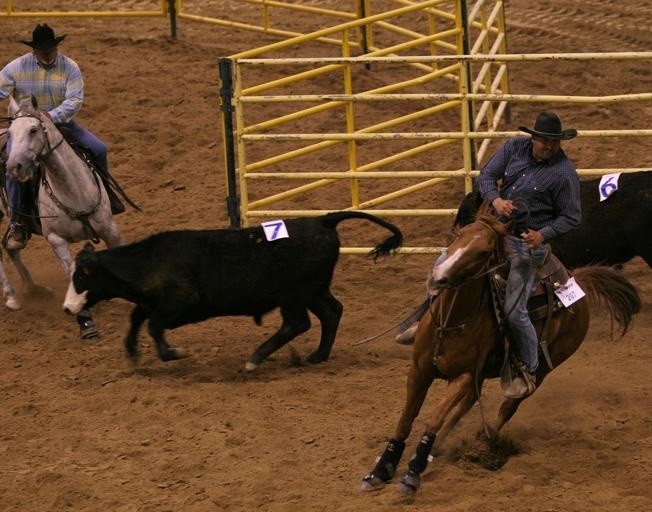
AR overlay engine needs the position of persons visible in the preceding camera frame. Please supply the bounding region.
[2,23,125,250]
[394,111,582,399]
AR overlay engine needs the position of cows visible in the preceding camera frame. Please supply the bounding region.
[452,171,652,284]
[60,210,403,372]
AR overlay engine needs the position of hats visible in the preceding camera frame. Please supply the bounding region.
[519,112,576,140]
[20,23,68,48]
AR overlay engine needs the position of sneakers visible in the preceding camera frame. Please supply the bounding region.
[504,374,537,398]
[111,200,125,214]
[396,324,418,343]
[6,230,32,250]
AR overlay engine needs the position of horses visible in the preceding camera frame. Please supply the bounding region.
[0,91,122,342]
[360,197,643,495]
